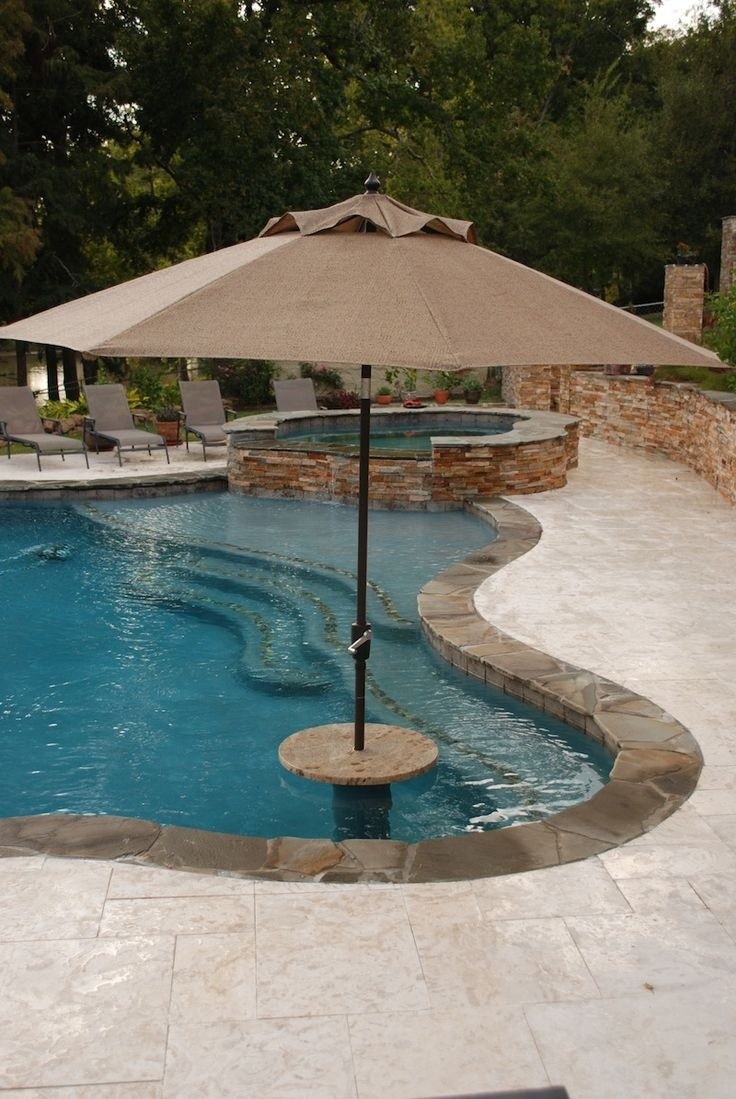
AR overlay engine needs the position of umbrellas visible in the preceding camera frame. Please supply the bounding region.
[0,171,732,753]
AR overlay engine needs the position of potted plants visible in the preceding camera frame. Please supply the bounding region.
[385,367,416,401]
[156,387,184,446]
[425,371,461,404]
[377,386,391,404]
[461,380,481,404]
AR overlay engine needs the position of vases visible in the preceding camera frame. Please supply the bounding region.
[83,420,113,451]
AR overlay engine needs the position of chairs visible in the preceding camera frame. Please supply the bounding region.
[0,386,90,471]
[273,378,327,411]
[83,385,170,468]
[179,380,237,462]
